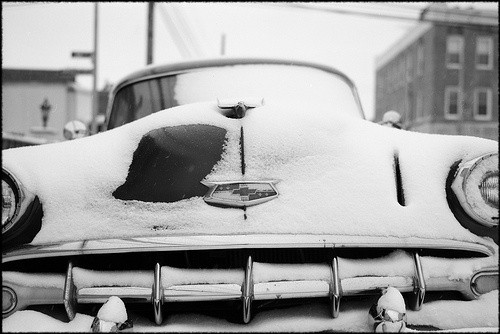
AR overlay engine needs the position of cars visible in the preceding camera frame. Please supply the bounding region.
[2,59,500,333]
